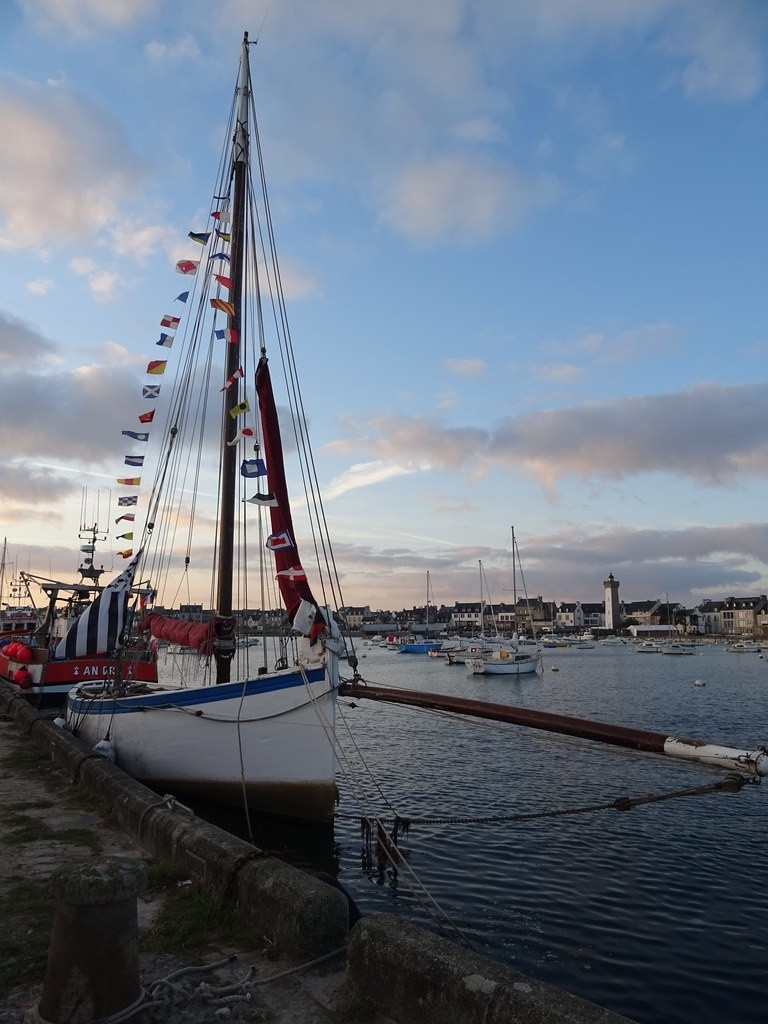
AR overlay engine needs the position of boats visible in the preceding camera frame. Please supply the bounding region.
[0,486,159,708]
[363,525,596,674]
[599,634,762,655]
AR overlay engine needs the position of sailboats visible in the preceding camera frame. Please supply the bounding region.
[60,26,363,831]
